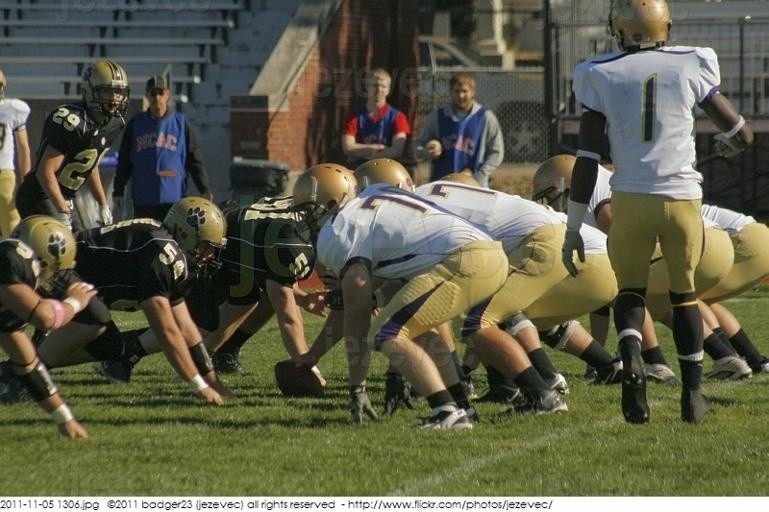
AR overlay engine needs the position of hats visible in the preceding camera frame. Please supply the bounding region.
[146,76,168,92]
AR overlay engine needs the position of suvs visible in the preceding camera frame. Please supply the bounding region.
[418,36,546,161]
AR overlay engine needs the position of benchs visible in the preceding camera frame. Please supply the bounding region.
[575,1,769,120]
[0,0,242,101]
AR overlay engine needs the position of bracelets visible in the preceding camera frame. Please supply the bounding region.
[721,115,747,137]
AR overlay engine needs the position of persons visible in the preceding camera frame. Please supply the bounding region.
[16,58,131,231]
[562,0,755,425]
[413,74,505,187]
[112,74,214,221]
[0,70,32,240]
[0,153,769,440]
[341,68,412,170]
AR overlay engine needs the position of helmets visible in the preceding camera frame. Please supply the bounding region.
[80,59,130,117]
[608,0,672,51]
[8,214,77,299]
[162,156,415,278]
[532,154,577,215]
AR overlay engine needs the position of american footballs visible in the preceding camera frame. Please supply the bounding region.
[275,360,323,393]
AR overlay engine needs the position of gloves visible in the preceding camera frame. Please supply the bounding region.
[96,205,113,227]
[562,227,585,278]
[713,132,741,158]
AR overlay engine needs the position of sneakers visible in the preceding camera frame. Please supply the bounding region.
[0,359,25,405]
[100,329,146,383]
[213,352,242,371]
